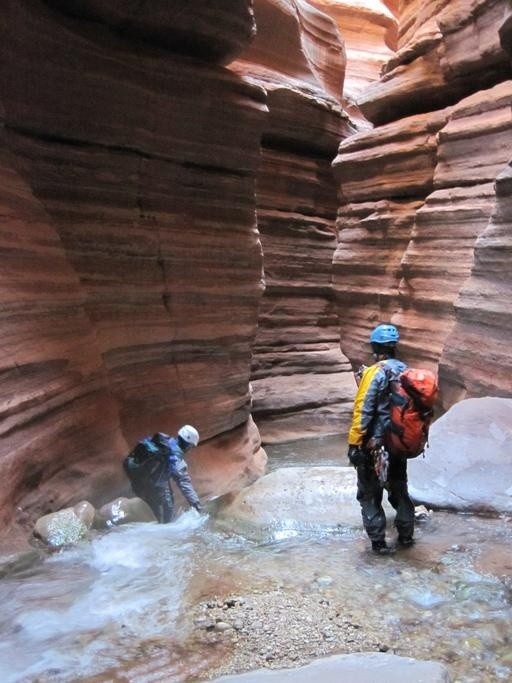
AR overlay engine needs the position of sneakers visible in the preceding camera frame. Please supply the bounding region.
[397,536,415,546]
[370,541,388,556]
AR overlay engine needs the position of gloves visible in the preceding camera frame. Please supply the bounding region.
[348,444,358,468]
[192,500,202,510]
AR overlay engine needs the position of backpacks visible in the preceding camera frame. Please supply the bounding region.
[377,362,438,459]
[122,432,184,485]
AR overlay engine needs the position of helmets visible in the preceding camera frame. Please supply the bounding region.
[178,425,200,447]
[370,324,400,344]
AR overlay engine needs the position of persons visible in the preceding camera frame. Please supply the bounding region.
[122,423,205,526]
[346,322,416,555]
